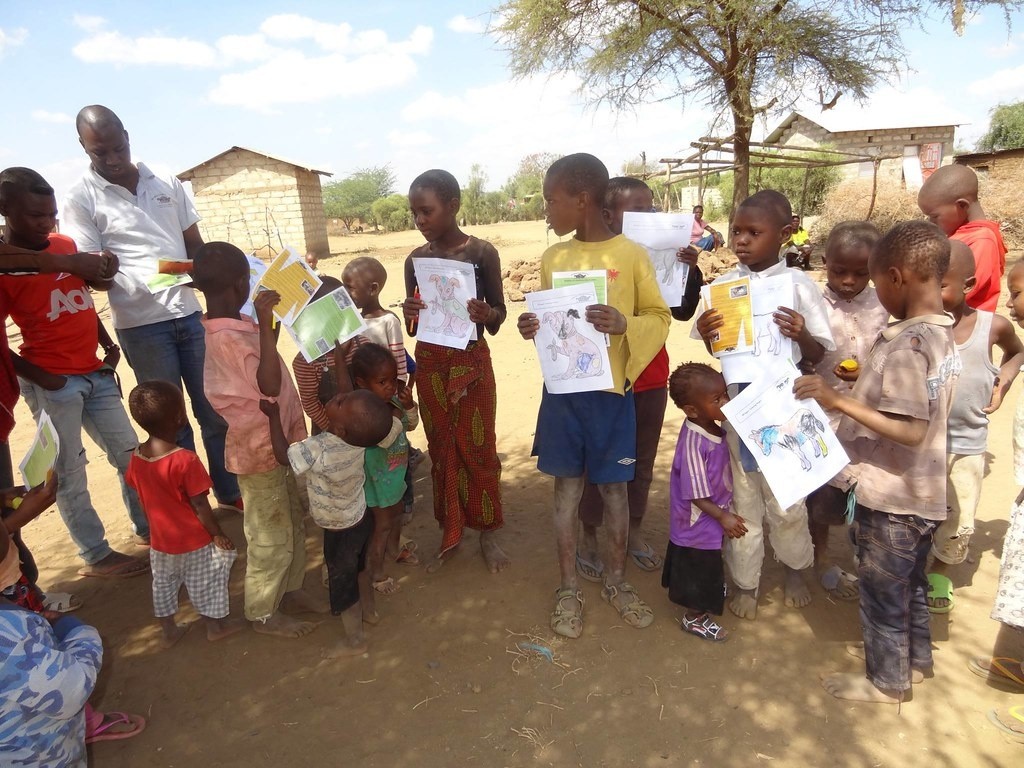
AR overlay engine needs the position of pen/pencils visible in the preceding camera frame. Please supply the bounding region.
[410,286,417,333]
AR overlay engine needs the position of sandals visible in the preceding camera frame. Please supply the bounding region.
[551,585,584,638]
[968,656,1024,689]
[600,581,653,629]
[681,610,730,643]
[987,704,1024,736]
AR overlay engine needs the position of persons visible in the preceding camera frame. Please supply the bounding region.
[662,363,748,642]
[917,164,1007,313]
[792,221,961,704]
[517,152,671,638]
[969,255,1024,688]
[925,239,1024,612]
[576,177,704,582]
[126,380,249,640]
[403,169,512,574]
[782,216,814,270]
[0,168,146,768]
[691,206,725,252]
[60,104,245,512]
[193,241,330,638]
[258,249,419,659]
[690,190,890,619]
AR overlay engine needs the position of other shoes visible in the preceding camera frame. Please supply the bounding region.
[218,496,244,513]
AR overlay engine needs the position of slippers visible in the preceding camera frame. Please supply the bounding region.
[575,550,605,582]
[628,539,661,571]
[383,548,418,565]
[77,555,151,578]
[41,591,82,611]
[84,711,145,742]
[925,573,954,613]
[134,532,151,548]
[373,571,401,597]
[812,557,862,601]
